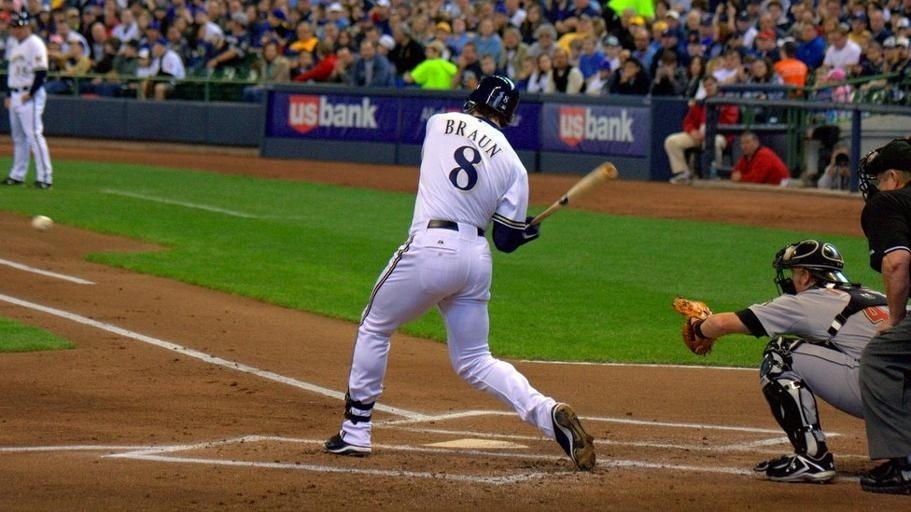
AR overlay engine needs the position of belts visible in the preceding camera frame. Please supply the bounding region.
[427,221,486,236]
[11,87,29,92]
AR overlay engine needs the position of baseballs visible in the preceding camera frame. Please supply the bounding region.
[31,215,53,233]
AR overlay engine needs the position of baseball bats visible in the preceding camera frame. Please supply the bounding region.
[528,163,617,227]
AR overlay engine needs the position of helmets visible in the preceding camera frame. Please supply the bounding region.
[462,75,520,122]
[858,138,910,203]
[773,240,848,296]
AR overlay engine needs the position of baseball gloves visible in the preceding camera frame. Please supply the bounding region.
[674,295,713,355]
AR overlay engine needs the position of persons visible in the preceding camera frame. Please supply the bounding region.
[675,241,900,486]
[853,135,910,493]
[0,0,911,118]
[816,148,854,192]
[6,16,56,186]
[733,129,789,186]
[323,74,605,470]
[663,76,740,187]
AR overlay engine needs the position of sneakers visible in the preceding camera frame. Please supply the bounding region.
[32,182,52,188]
[669,173,694,184]
[320,433,372,456]
[4,179,23,185]
[753,450,836,481]
[860,461,911,495]
[552,403,596,471]
